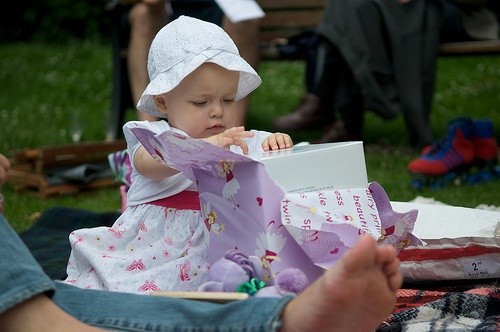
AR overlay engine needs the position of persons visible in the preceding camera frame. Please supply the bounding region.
[274,0,500,149]
[107,0,262,131]
[0,151,404,332]
[69,15,294,300]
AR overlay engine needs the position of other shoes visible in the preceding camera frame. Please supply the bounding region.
[315,120,361,144]
[274,92,338,130]
[409,116,498,193]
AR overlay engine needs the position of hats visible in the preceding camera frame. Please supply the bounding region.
[135,13,261,118]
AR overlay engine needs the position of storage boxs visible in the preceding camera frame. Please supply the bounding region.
[200,140,369,287]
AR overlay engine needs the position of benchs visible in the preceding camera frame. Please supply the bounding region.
[258,0,500,62]
